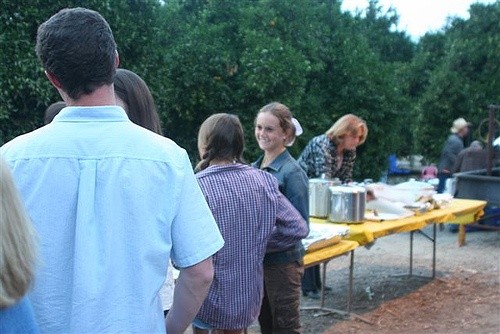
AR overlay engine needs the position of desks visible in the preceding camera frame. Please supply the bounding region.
[310,198,487,324]
[303,240,358,268]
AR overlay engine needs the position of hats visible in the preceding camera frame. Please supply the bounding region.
[449,118,471,134]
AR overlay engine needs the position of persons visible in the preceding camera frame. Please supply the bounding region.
[298,114,368,298]
[172,114,309,333]
[247,102,309,334]
[0,9,225,334]
[437,118,490,232]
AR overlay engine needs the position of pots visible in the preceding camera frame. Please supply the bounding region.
[308,177,366,224]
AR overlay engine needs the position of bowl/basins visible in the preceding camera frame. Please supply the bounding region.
[396,179,435,205]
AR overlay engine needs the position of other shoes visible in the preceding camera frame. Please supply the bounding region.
[319,284,332,292]
[302,287,325,299]
